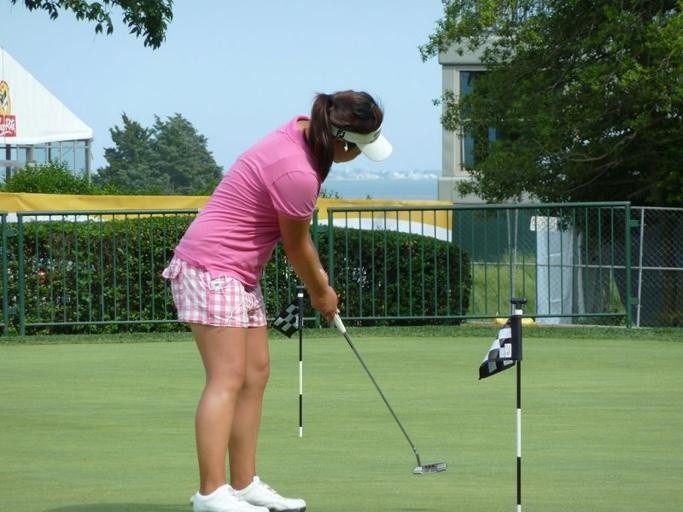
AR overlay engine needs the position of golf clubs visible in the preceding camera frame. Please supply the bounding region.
[333,312,447,475]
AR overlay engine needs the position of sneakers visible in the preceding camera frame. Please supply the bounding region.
[193,484,269,512]
[236,475,307,511]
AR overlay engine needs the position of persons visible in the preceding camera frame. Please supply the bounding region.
[160,90,383,512]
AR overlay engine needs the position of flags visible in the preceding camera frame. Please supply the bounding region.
[270,297,304,338]
[478,314,521,381]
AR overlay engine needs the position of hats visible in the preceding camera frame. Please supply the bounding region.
[327,122,392,160]
